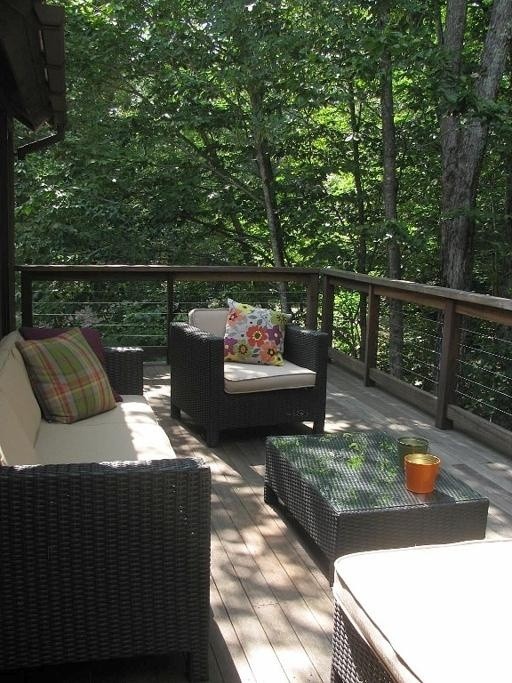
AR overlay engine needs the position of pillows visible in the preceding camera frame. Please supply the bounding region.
[219,296,293,366]
[15,328,125,425]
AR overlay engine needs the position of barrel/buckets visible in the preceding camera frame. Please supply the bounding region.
[404,452,440,493]
[398,436,429,466]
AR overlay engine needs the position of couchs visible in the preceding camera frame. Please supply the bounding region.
[0,327,212,682]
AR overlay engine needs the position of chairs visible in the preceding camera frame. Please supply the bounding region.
[165,301,332,449]
